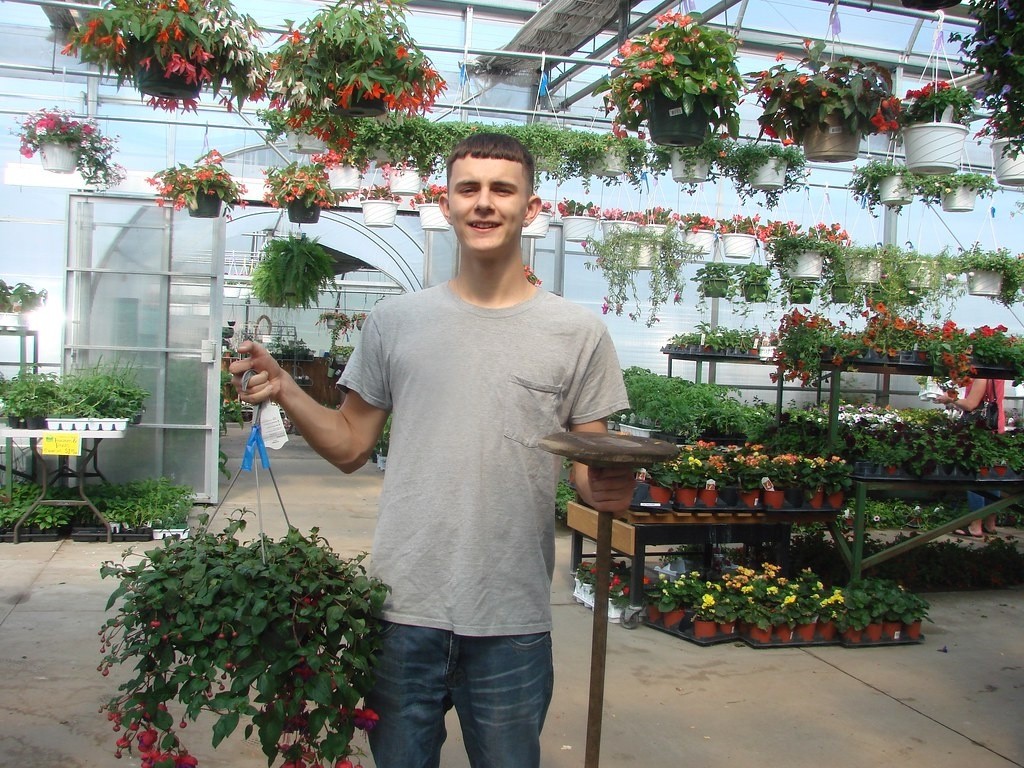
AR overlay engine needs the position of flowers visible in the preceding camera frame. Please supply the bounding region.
[97,505,392,767]
[58,1,1024,398]
[241,400,253,412]
[573,526,1024,632]
[0,280,49,313]
[839,498,1024,530]
[638,398,1024,502]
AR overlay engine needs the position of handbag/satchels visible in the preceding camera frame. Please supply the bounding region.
[961,400,999,431]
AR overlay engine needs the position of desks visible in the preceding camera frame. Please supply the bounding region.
[1,429,126,544]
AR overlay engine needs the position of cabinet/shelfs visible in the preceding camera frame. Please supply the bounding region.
[659,347,1024,581]
[238,323,297,412]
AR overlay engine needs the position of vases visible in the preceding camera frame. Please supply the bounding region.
[649,485,844,507]
[789,285,814,305]
[601,220,640,242]
[588,146,626,175]
[980,466,991,477]
[287,194,320,224]
[906,515,925,525]
[337,88,388,116]
[648,93,715,147]
[670,151,709,182]
[847,519,854,526]
[639,224,666,238]
[535,148,559,171]
[521,212,552,239]
[0,313,30,330]
[417,204,452,231]
[990,138,1024,186]
[356,320,371,332]
[241,412,252,421]
[865,289,890,308]
[1007,515,1017,524]
[748,156,789,189]
[843,257,884,283]
[389,167,420,195]
[905,261,940,290]
[679,345,687,352]
[941,183,979,211]
[286,125,327,153]
[135,39,203,100]
[680,230,716,254]
[703,344,711,352]
[623,238,663,270]
[878,175,914,204]
[751,349,759,355]
[967,270,1003,295]
[328,319,337,329]
[994,464,1008,476]
[327,163,364,189]
[885,463,896,475]
[228,321,234,326]
[334,355,347,366]
[574,577,921,643]
[900,123,970,174]
[222,285,240,297]
[915,350,935,363]
[373,148,394,162]
[40,141,80,173]
[361,201,399,227]
[239,287,259,299]
[804,111,861,163]
[784,249,827,279]
[832,285,854,304]
[561,216,597,242]
[821,345,830,353]
[189,193,222,218]
[721,233,762,258]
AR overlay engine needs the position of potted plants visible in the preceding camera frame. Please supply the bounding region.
[734,262,778,330]
[610,365,773,446]
[690,264,745,324]
[0,351,150,431]
[0,475,198,543]
[371,413,392,471]
[251,231,338,311]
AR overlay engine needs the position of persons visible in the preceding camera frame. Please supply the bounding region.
[933,377,1007,538]
[227,129,641,768]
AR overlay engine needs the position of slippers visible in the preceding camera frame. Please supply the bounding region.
[981,523,998,537]
[948,526,985,542]
